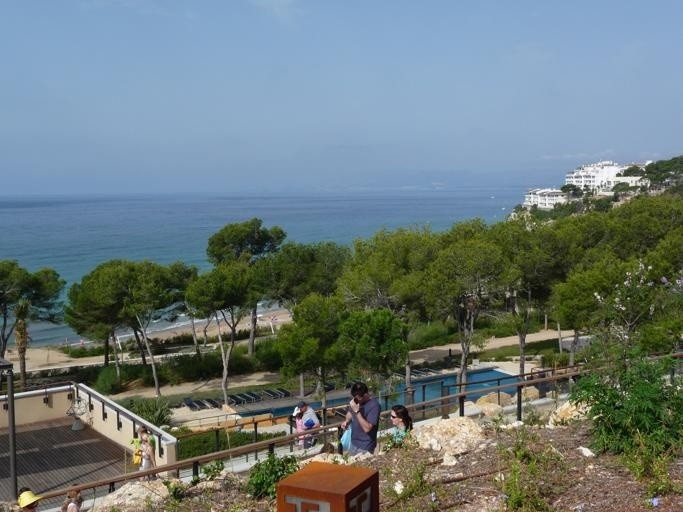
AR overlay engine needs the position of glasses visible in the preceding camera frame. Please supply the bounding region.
[391,415,399,418]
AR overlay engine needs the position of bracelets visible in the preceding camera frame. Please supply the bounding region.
[354,410,360,414]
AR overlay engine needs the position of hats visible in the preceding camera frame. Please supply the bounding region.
[18,491,44,509]
[292,407,302,417]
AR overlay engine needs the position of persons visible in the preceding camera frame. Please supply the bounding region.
[18,489,43,512]
[340,381,381,456]
[387,404,413,447]
[128,425,159,481]
[291,404,305,448]
[298,401,321,448]
[60,487,92,512]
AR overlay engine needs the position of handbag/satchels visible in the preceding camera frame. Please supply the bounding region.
[340,423,351,451]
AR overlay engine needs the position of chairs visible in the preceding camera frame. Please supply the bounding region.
[181,387,291,411]
